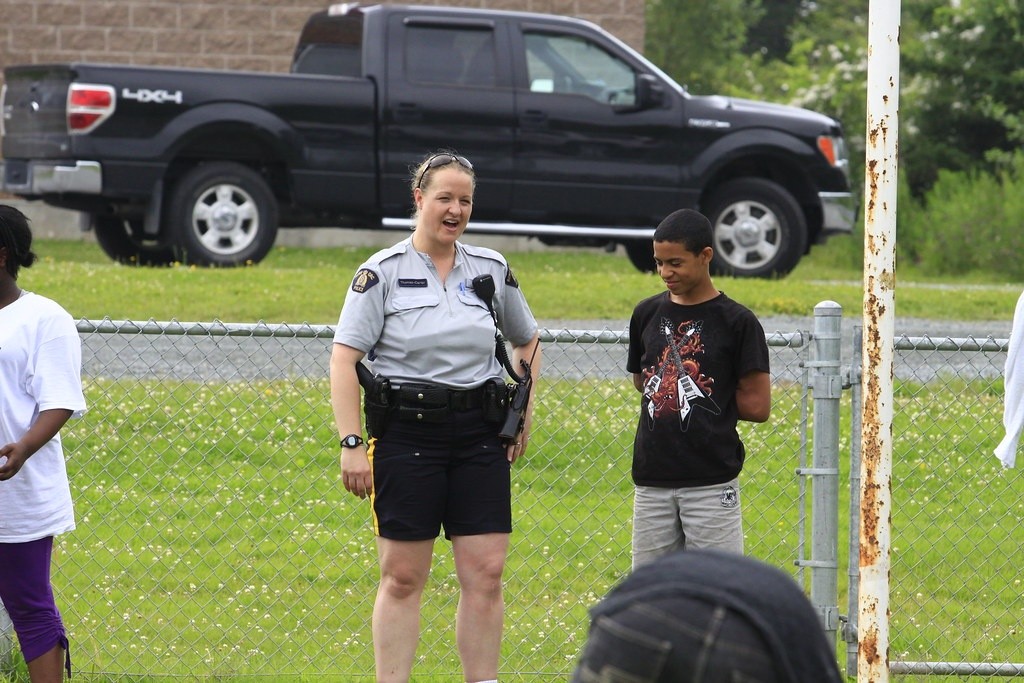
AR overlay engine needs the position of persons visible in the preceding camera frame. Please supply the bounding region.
[327,150,544,683]
[626,209,771,572]
[567,544,844,682]
[0,200,87,683]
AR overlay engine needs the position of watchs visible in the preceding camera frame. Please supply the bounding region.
[340,433,363,448]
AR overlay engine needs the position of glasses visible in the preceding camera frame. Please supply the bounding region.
[418,153,474,188]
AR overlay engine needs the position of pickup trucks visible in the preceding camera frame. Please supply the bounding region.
[0,1,862,284]
